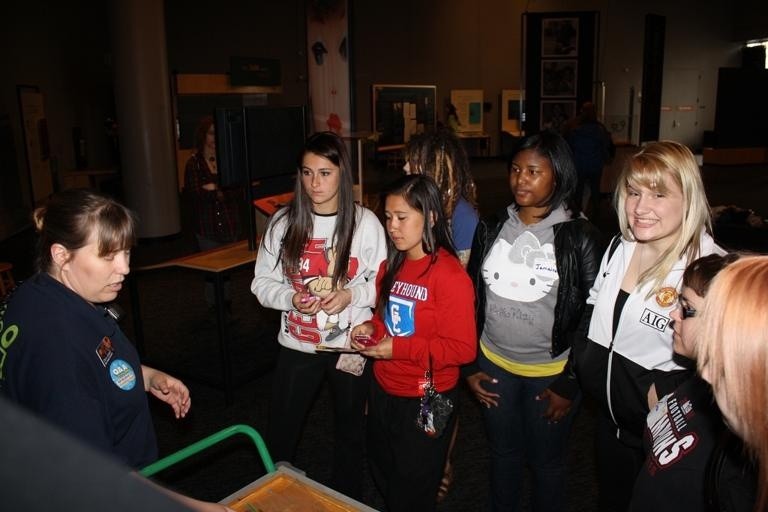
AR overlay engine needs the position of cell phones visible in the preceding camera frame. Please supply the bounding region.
[300,295,322,305]
[355,334,378,348]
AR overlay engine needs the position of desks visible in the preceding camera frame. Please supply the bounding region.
[127,235,262,408]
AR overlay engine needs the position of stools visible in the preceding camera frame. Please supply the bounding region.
[0,262,16,299]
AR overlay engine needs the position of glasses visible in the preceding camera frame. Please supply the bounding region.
[674,289,698,321]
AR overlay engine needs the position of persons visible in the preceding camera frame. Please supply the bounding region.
[0,188,191,470]
[184,118,248,324]
